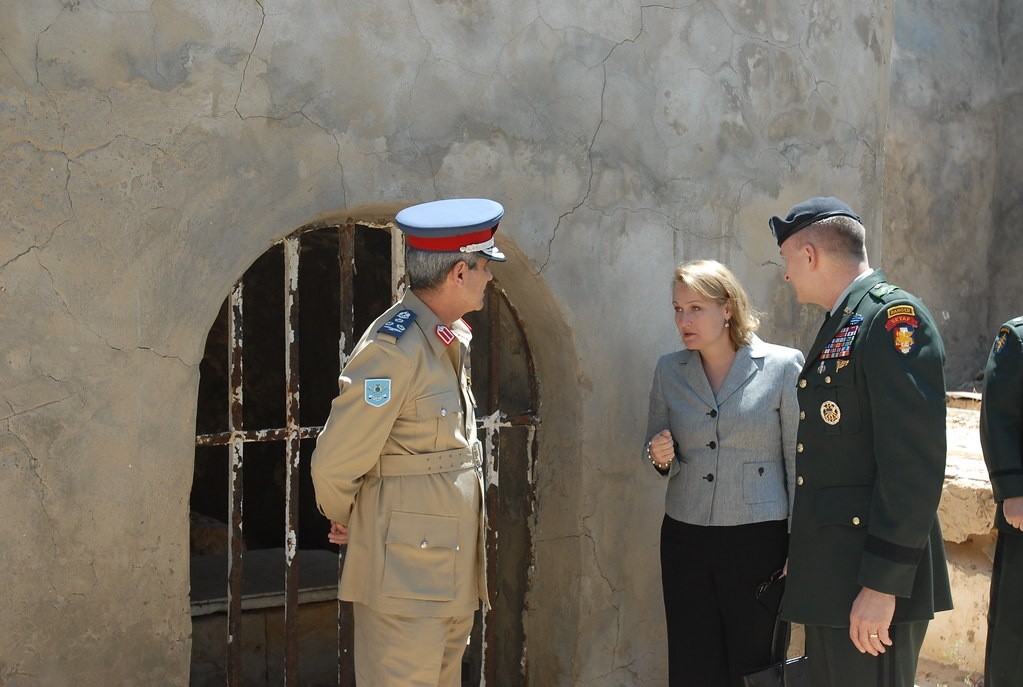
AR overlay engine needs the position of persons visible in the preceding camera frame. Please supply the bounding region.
[979,313,1023,687]
[308,198,508,687]
[768,194,954,687]
[640,257,808,686]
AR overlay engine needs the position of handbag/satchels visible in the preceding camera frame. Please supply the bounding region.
[742,598,810,687]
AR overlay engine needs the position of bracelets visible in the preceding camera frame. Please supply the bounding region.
[646,441,671,468]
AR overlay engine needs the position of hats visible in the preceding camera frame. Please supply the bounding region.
[768,196,863,246]
[394,198,509,263]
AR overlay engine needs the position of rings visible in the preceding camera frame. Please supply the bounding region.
[869,633,877,639]
